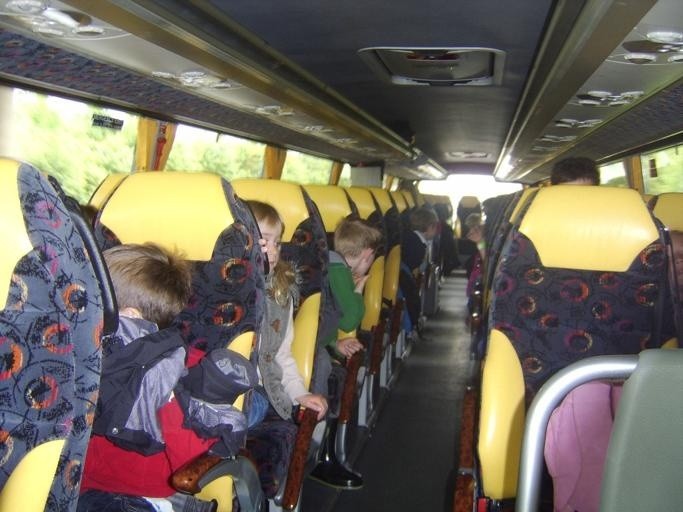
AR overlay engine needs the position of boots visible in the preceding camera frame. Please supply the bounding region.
[306,411,365,492]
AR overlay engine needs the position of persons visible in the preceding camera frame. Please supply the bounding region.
[465,157,676,512]
[85,178,460,512]
